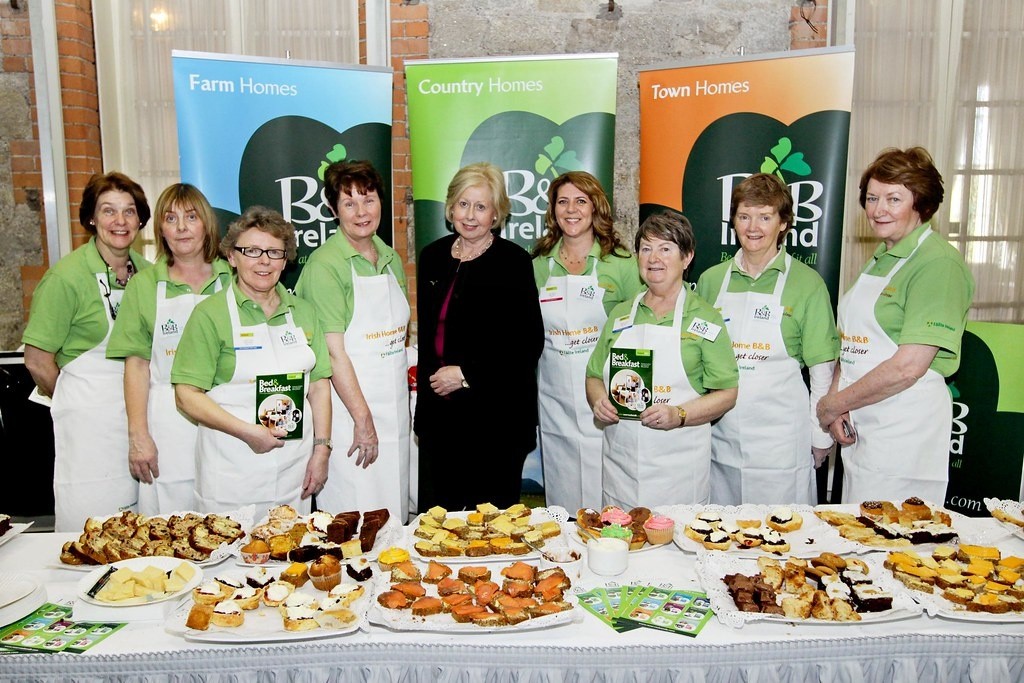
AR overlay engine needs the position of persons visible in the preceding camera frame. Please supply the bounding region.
[413,162,545,516]
[21,170,154,533]
[692,172,841,507]
[105,183,233,518]
[294,159,412,526]
[170,206,334,529]
[529,171,646,522]
[816,146,975,507]
[585,209,739,513]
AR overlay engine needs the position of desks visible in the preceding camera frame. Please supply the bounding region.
[0,517,1024,683]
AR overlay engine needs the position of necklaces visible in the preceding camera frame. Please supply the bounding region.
[561,251,587,264]
[455,234,493,259]
[108,261,132,286]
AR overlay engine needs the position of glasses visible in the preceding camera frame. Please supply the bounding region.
[232,245,287,258]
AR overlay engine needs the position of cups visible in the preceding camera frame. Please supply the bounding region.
[540,547,583,581]
[587,538,629,576]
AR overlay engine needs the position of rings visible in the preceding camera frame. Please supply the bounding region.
[657,420,659,424]
[360,450,365,452]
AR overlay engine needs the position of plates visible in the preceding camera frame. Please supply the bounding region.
[75,555,204,607]
[570,532,663,552]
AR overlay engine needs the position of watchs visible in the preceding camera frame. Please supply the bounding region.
[461,371,470,389]
[313,439,333,450]
[675,406,687,426]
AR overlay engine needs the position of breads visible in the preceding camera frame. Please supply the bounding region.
[0,514,10,537]
[57,502,571,633]
[683,496,1024,621]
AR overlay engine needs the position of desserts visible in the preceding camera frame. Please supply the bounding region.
[576,505,677,550]
